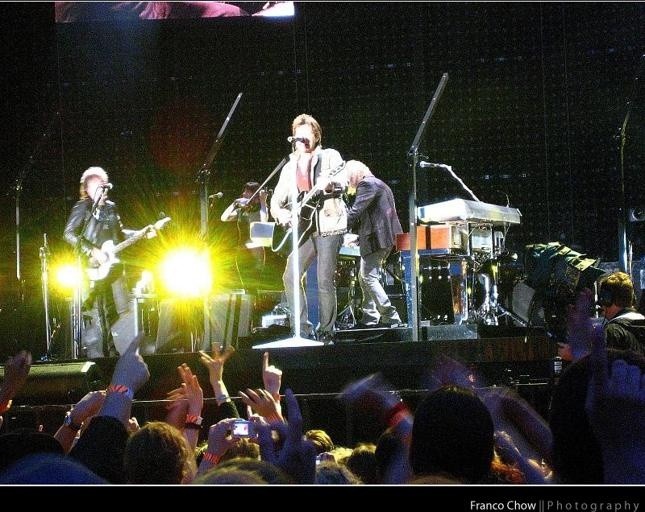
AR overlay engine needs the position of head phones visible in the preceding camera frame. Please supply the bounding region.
[599,273,623,307]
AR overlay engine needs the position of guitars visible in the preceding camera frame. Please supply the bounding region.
[85,217,171,281]
[271,160,347,259]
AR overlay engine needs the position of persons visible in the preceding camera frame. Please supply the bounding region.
[269,113,348,341]
[0,342,643,486]
[62,165,156,357]
[220,182,276,329]
[342,159,404,327]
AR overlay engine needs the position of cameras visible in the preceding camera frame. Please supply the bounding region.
[231,418,254,437]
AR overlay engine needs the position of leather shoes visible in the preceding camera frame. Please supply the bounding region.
[300,333,333,342]
[346,319,402,330]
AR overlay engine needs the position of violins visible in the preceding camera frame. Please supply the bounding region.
[243,189,273,213]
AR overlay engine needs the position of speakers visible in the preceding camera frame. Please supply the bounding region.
[0,361,106,405]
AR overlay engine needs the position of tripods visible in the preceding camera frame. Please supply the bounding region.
[338,261,363,327]
[469,259,529,328]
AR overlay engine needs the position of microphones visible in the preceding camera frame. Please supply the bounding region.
[208,192,223,199]
[100,183,112,189]
[420,161,448,168]
[288,137,309,145]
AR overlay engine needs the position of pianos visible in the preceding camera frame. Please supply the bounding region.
[417,198,521,227]
